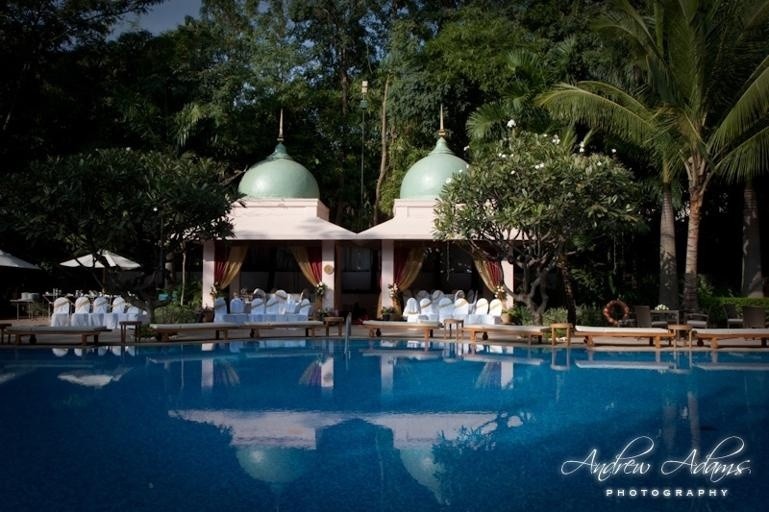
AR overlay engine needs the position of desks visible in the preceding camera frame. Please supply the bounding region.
[651,310,679,328]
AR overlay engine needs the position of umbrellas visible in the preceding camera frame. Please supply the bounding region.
[59,247,143,300]
[0,249,42,271]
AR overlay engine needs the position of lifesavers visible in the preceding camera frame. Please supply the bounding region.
[603,300,630,325]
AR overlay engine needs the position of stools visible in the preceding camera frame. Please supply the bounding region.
[687,320,708,329]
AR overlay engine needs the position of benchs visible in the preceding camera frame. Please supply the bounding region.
[242,320,324,338]
[149,323,241,340]
[4,326,107,346]
[692,328,769,349]
[574,325,673,348]
[462,324,550,345]
[363,320,442,339]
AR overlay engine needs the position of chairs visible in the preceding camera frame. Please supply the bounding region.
[213,288,311,321]
[722,304,743,329]
[9,289,149,331]
[682,303,713,330]
[400,289,503,325]
[741,306,769,340]
[634,305,669,341]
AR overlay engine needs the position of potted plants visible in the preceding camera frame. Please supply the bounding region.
[380,306,395,321]
